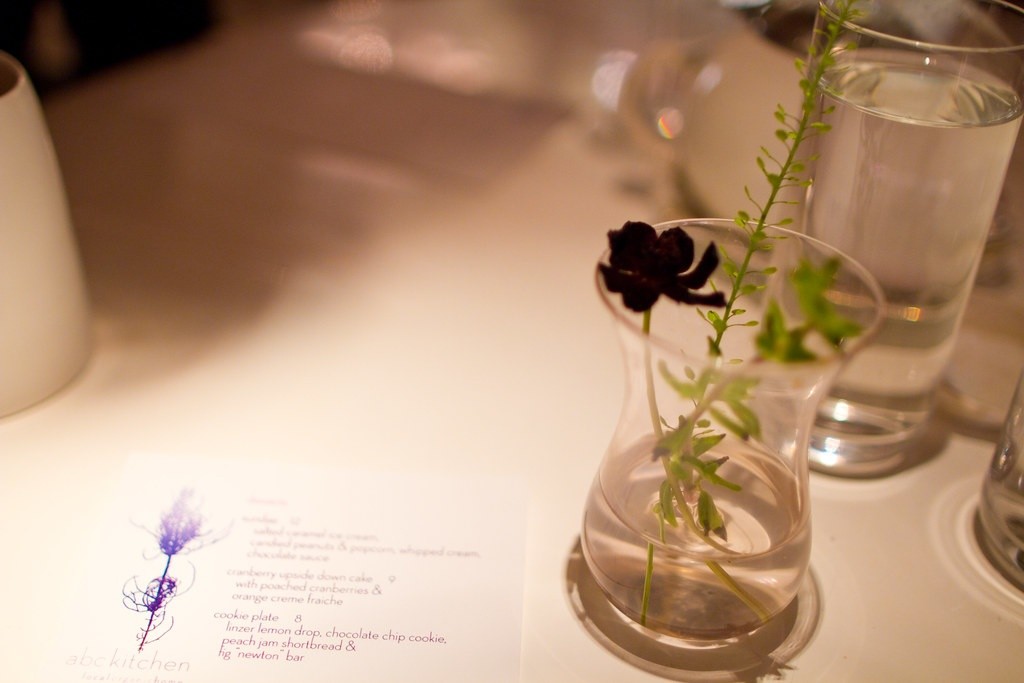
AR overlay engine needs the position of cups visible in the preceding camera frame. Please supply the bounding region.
[0,49,92,419]
[680,0,1024,617]
[564,220,886,670]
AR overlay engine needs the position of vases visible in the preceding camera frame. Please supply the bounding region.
[565,218,888,680]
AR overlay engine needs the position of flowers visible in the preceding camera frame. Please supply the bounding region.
[600,0,866,622]
